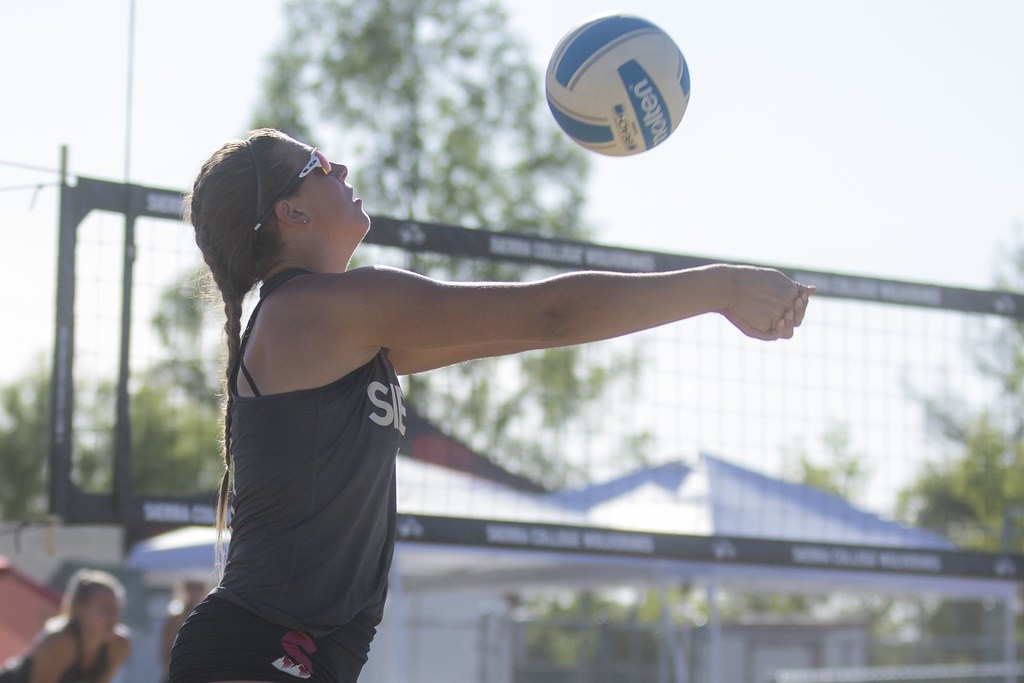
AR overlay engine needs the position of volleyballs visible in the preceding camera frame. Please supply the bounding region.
[545,12,690,157]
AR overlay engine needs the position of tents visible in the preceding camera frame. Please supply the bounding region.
[124,450,1024,682]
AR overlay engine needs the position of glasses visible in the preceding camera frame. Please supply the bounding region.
[254,146,331,231]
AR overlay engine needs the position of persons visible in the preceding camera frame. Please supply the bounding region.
[0,566,132,683]
[168,127,817,683]
[158,572,209,672]
